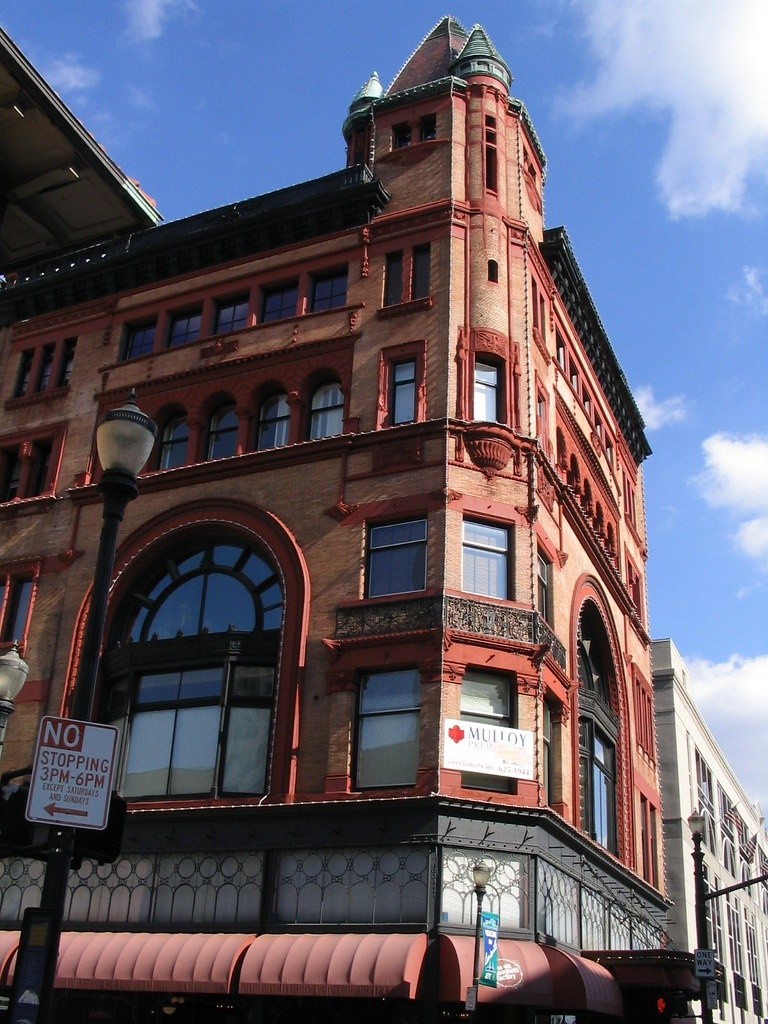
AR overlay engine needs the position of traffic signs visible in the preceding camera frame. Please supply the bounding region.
[24,714,120,832]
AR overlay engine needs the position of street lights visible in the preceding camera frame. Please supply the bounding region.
[466,856,492,1024]
[688,809,713,1024]
[0,384,160,1023]
